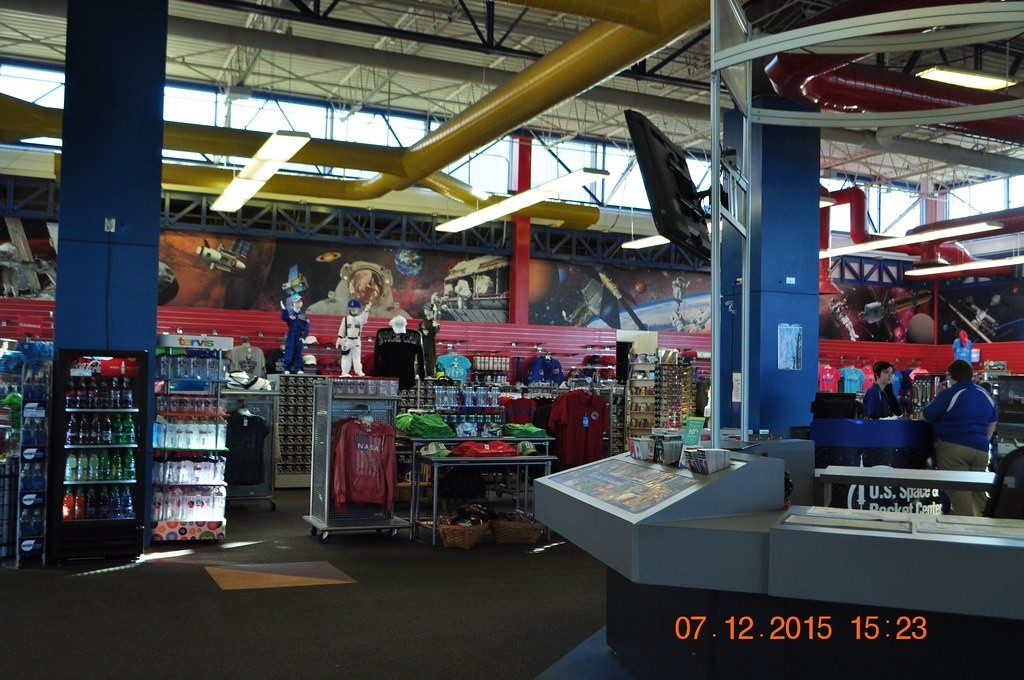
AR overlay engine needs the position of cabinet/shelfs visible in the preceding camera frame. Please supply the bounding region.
[15,359,52,569]
[268,373,329,488]
[629,367,661,435]
[153,333,234,542]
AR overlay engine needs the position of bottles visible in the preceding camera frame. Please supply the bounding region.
[18,362,44,537]
[62,377,135,519]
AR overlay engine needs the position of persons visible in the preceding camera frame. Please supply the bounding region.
[374,315,427,391]
[922,360,998,519]
[280,295,311,375]
[336,299,373,377]
[861,362,904,419]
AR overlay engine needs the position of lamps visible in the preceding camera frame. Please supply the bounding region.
[209,131,312,213]
[916,65,1016,92]
[436,165,610,236]
[904,254,1024,279]
[620,219,724,249]
[818,220,1002,262]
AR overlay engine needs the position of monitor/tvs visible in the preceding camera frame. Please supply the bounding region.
[623,108,712,261]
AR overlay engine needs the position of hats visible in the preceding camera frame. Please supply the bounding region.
[318,336,334,346]
[602,368,615,377]
[303,355,317,366]
[348,300,362,309]
[319,357,332,366]
[305,336,319,345]
[389,316,407,333]
[604,356,616,365]
[587,356,600,363]
[515,441,538,456]
[959,330,968,346]
[420,442,452,457]
[291,295,304,305]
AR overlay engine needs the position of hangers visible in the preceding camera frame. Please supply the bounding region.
[499,378,609,400]
[820,357,925,367]
[231,399,267,421]
[235,334,289,347]
[439,340,556,358]
[345,405,386,424]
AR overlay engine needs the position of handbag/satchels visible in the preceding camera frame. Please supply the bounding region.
[339,337,350,351]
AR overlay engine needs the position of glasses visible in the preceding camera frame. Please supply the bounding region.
[653,366,697,428]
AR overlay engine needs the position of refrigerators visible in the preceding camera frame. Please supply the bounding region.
[48,348,148,560]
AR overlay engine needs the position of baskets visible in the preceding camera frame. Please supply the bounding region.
[490,519,545,544]
[437,518,489,549]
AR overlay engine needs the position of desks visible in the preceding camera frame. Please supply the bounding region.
[413,454,558,546]
[398,435,555,544]
